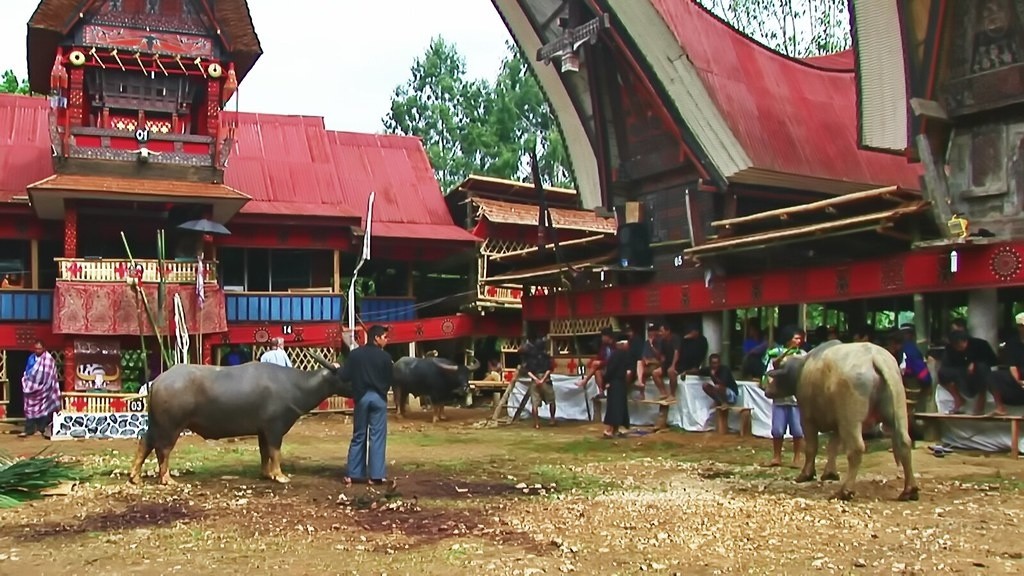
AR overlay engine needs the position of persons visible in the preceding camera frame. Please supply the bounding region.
[574,321,738,438]
[339,325,393,485]
[1,274,16,289]
[426,350,447,423]
[525,339,556,429]
[17,341,61,439]
[474,352,502,380]
[761,329,808,469]
[221,338,292,368]
[740,318,1024,415]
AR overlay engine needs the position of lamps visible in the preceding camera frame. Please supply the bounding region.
[948,213,968,237]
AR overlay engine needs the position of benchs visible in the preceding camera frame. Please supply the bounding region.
[914,413,1024,461]
[715,406,754,437]
[591,398,677,428]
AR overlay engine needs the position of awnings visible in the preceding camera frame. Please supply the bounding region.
[682,185,933,257]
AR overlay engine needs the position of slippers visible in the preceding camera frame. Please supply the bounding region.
[934,449,945,456]
[928,446,953,452]
[944,408,965,414]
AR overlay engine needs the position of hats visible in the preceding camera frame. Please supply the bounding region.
[623,321,632,330]
[1015,312,1024,325]
[900,323,915,333]
[648,321,657,330]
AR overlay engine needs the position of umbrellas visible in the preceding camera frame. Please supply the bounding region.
[176,219,231,235]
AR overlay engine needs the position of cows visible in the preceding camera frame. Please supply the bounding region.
[129,349,354,486]
[762,340,920,502]
[390,356,481,423]
